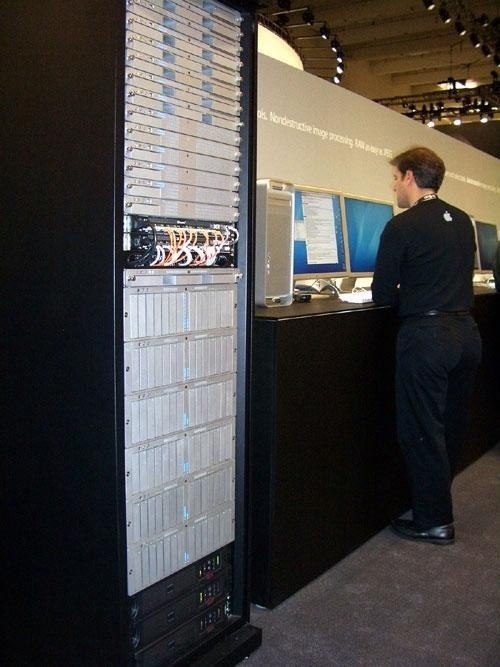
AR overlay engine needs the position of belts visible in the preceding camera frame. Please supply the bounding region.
[425,309,469,315]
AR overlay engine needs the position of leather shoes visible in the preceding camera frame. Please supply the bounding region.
[389,518,455,545]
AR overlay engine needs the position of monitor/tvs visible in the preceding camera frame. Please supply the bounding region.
[293,183,348,300]
[473,219,499,273]
[342,192,396,293]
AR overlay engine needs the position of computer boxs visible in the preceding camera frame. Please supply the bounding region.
[255,177,295,309]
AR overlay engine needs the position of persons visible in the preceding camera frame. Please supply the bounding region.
[369,146,486,547]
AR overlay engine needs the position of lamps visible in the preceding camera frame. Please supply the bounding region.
[258,0,500,128]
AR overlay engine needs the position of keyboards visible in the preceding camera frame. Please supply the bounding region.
[338,291,374,304]
